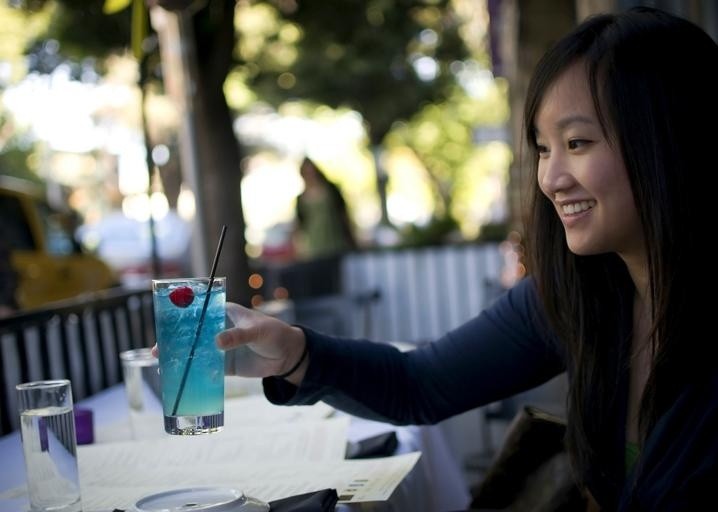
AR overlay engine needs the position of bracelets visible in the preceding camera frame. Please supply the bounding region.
[278,324,307,380]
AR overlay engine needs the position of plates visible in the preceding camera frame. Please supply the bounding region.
[129,483,271,512]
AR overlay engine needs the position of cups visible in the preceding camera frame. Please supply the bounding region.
[119,348,163,441]
[15,379,83,510]
[152,279,226,435]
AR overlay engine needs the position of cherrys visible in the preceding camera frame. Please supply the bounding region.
[170,286,194,307]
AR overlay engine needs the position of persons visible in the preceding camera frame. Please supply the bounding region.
[152,8,718,512]
[299,157,359,272]
